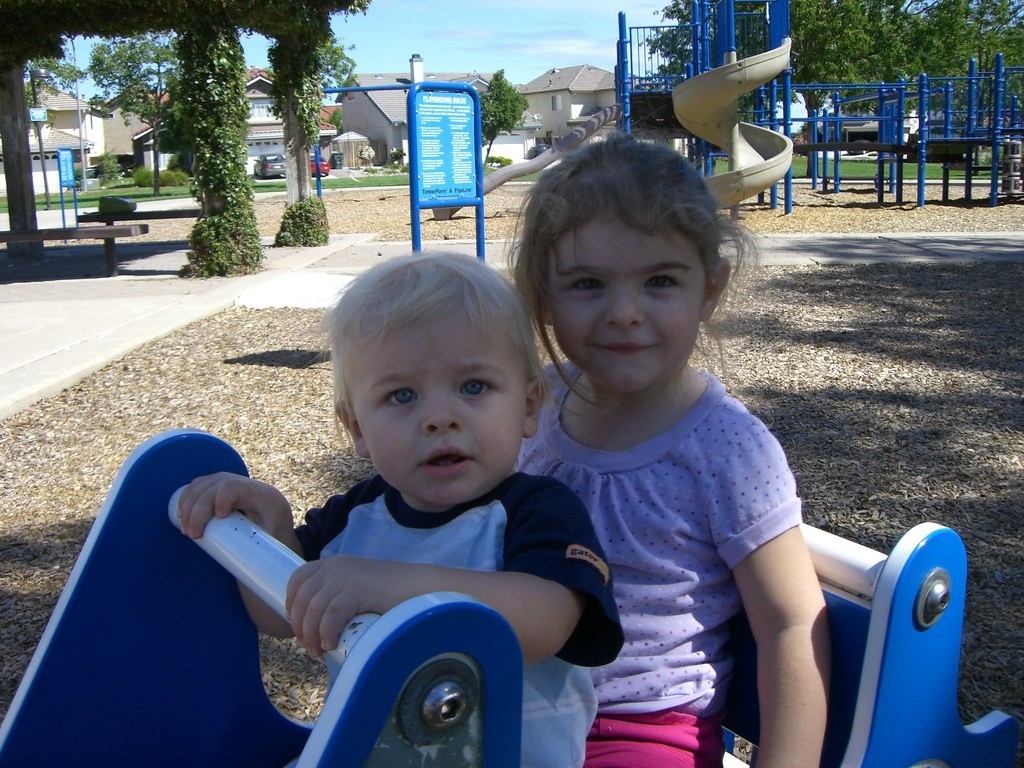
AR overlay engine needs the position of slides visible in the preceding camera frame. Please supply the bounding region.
[671,36,793,209]
[430,101,622,208]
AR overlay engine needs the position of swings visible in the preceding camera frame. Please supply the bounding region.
[345,92,371,183]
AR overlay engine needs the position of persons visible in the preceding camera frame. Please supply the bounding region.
[510,142,837,768]
[175,248,624,768]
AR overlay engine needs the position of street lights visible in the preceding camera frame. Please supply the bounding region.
[29,69,53,210]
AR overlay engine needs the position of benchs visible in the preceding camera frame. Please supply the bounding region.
[78,206,204,226]
[0,224,149,278]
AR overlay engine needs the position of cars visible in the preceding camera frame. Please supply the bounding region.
[309,153,330,175]
[535,144,549,158]
[254,154,286,177]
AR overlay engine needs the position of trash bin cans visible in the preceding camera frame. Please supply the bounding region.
[332,151,344,170]
[535,144,550,156]
